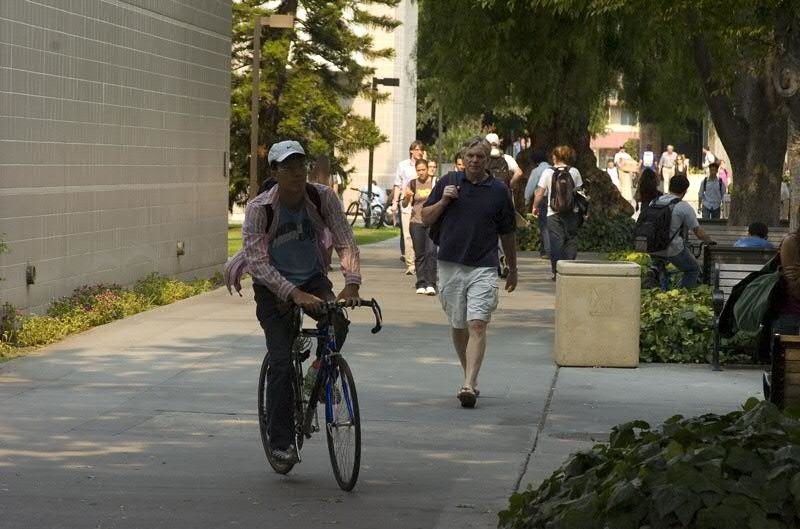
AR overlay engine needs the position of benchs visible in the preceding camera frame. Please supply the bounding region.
[713,262,767,372]
[722,193,731,219]
[762,331,800,412]
[704,246,780,287]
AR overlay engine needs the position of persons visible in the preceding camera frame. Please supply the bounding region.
[486,134,522,277]
[421,136,518,408]
[648,175,712,288]
[734,222,774,248]
[761,207,800,366]
[362,180,384,225]
[607,145,689,211]
[698,163,727,219]
[392,141,464,294]
[702,146,731,191]
[525,145,583,280]
[224,141,362,462]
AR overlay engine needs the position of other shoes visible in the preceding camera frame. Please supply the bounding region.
[416,288,425,294]
[399,255,406,263]
[425,286,436,295]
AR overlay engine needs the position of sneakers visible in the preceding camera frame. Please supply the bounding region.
[271,447,297,466]
[303,368,341,404]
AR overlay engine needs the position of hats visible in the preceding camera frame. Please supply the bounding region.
[486,133,499,146]
[268,140,305,167]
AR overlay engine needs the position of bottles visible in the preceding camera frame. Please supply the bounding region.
[302,360,320,397]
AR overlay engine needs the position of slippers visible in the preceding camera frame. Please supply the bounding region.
[458,386,476,408]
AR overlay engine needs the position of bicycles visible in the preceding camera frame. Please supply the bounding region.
[345,187,385,230]
[641,240,718,292]
[257,296,385,491]
[381,202,402,229]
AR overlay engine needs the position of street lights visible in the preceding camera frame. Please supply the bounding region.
[364,76,400,227]
[249,13,295,200]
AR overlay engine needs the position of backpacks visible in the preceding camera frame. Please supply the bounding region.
[485,153,508,186]
[633,198,684,252]
[548,166,576,211]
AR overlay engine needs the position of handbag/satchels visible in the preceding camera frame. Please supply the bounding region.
[718,252,785,336]
[427,170,457,245]
